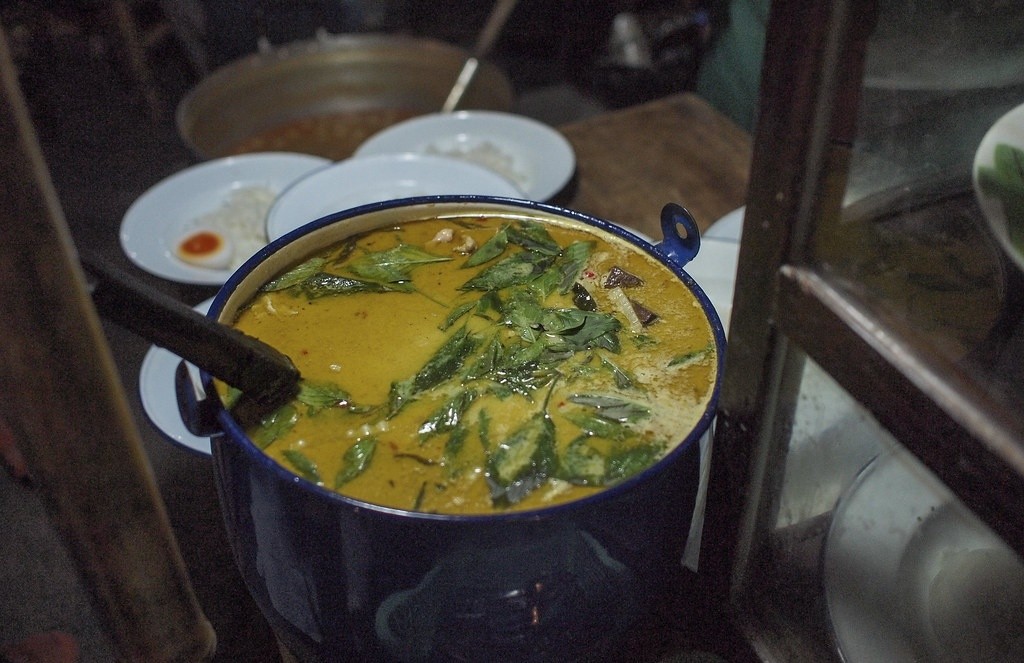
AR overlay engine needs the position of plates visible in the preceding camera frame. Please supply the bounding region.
[973,102,1024,272]
[120,109,746,456]
[822,440,1024,663]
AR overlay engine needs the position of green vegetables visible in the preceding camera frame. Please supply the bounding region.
[223,218,716,511]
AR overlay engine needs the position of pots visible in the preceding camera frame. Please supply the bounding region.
[175,200,726,663]
[173,33,521,163]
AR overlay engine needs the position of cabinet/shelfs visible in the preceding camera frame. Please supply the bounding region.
[690,2,1024,662]
